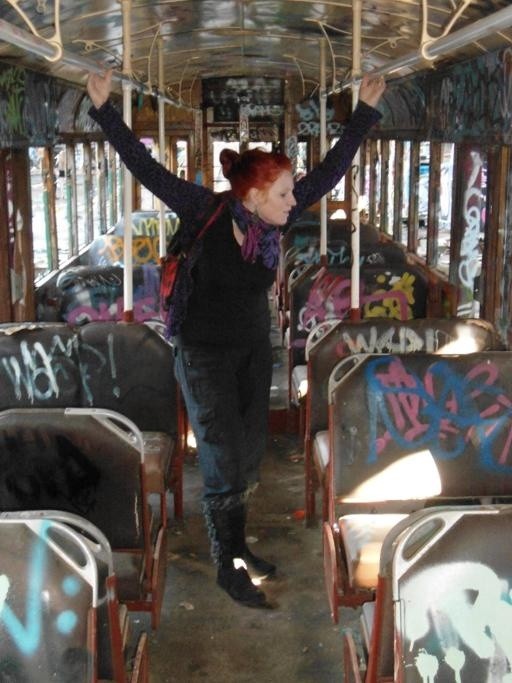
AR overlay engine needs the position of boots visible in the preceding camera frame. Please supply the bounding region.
[202,493,266,605]
[231,481,276,580]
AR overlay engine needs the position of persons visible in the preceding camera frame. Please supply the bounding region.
[85,68,388,607]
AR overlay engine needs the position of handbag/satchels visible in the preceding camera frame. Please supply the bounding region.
[159,197,226,323]
